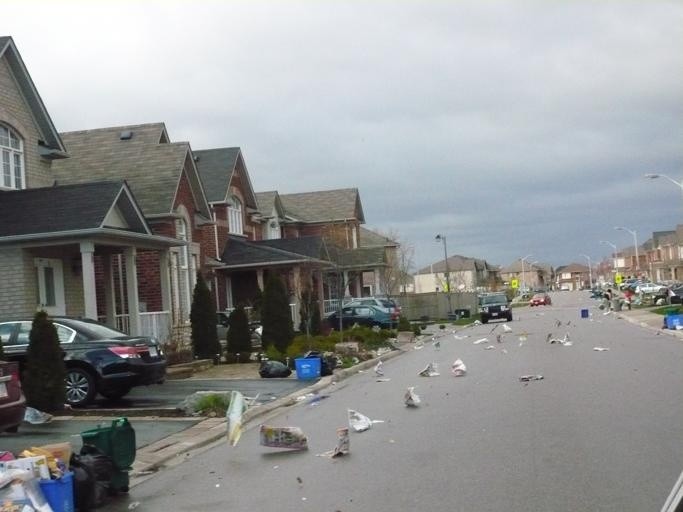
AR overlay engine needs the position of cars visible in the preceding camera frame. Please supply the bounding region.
[603,279,683,295]
[529,292,552,307]
[213,309,265,352]
[321,305,400,333]
[0,358,27,438]
[0,315,169,409]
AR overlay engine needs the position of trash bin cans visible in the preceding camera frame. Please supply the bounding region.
[80,418,136,492]
[39,470,75,512]
[294,357,321,379]
[581,309,588,318]
[664,313,683,329]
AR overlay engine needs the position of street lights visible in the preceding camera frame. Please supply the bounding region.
[435,231,457,321]
[614,226,641,277]
[599,240,618,275]
[577,253,593,292]
[520,252,539,298]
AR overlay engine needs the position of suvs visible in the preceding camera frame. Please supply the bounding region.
[341,297,402,322]
[479,294,514,324]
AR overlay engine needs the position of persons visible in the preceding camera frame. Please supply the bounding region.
[602,288,612,314]
[623,286,633,310]
